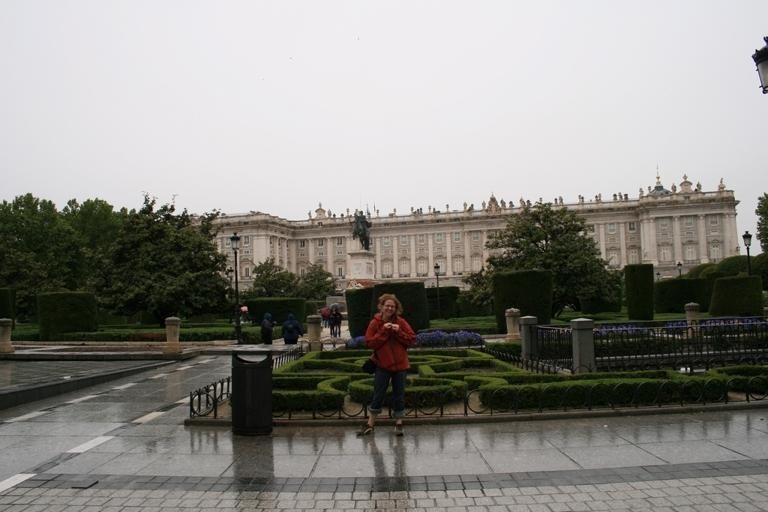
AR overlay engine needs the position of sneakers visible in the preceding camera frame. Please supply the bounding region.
[357,424,374,435]
[394,424,403,436]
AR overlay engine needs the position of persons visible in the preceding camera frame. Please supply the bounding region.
[350,210,372,235]
[260,313,277,345]
[319,302,344,339]
[281,313,304,344]
[355,294,417,438]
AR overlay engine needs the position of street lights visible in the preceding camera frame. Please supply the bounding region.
[677,262,682,277]
[743,231,752,276]
[434,263,442,318]
[230,231,242,340]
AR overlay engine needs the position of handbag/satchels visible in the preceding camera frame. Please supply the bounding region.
[362,353,376,373]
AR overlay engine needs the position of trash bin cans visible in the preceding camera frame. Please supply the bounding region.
[231,352,272,435]
